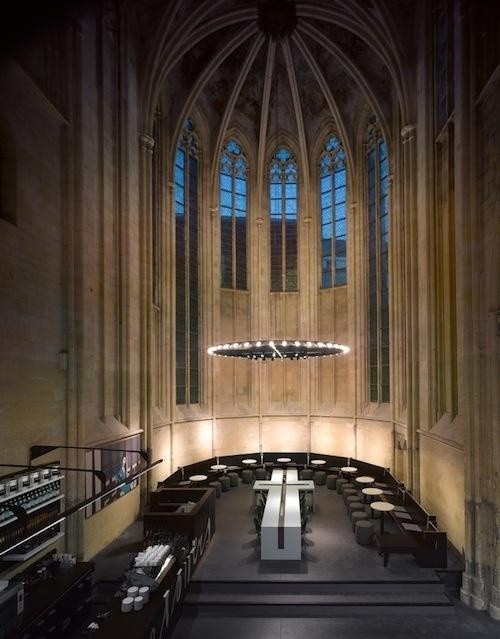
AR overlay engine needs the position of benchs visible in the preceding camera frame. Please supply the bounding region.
[154,452,449,570]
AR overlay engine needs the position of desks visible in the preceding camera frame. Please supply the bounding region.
[362,487,383,520]
[277,458,291,469]
[242,459,258,469]
[341,466,358,483]
[311,460,326,471]
[211,465,227,473]
[370,502,395,535]
[82,534,193,639]
[0,562,96,639]
[189,474,207,488]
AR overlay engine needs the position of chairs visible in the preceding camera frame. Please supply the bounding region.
[253,491,309,547]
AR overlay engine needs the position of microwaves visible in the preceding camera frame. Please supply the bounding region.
[0,582,25,623]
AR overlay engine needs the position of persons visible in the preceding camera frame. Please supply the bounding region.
[105,455,141,494]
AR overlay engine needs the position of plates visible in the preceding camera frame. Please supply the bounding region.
[121,586,151,614]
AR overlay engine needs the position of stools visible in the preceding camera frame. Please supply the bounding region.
[209,469,314,498]
[315,471,373,546]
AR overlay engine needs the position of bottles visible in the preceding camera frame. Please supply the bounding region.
[0,480,62,525]
